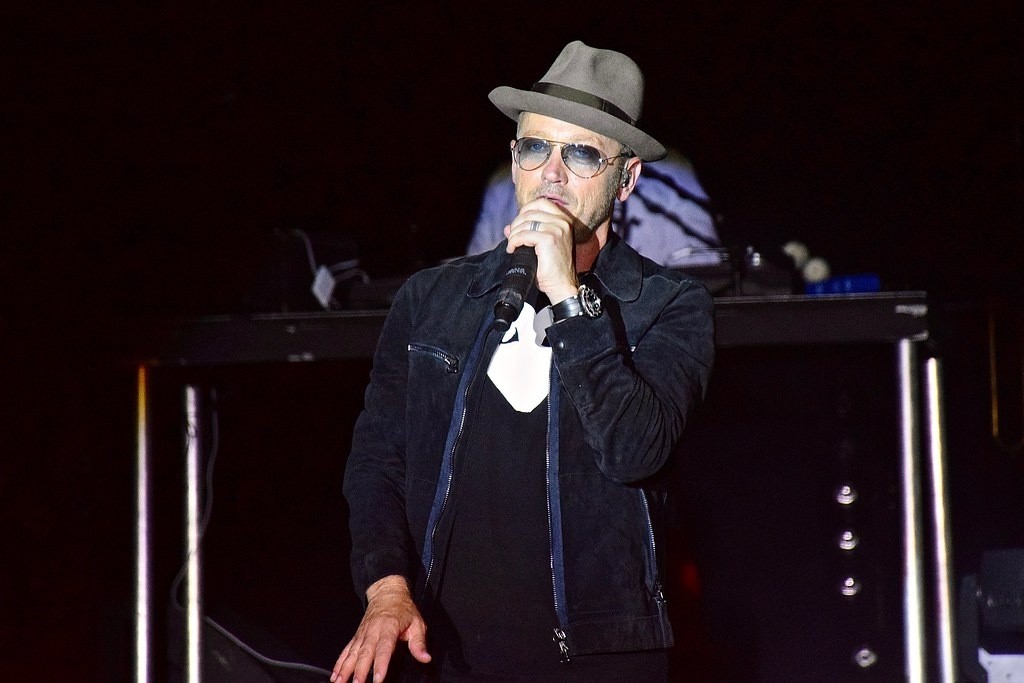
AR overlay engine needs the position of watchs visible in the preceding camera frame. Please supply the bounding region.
[547,284,604,324]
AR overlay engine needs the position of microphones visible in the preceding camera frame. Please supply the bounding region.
[492,245,538,332]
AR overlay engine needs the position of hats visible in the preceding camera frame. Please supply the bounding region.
[489,40,668,163]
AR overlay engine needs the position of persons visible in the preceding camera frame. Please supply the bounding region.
[329,39,721,683]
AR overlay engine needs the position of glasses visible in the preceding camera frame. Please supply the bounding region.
[509,137,629,179]
